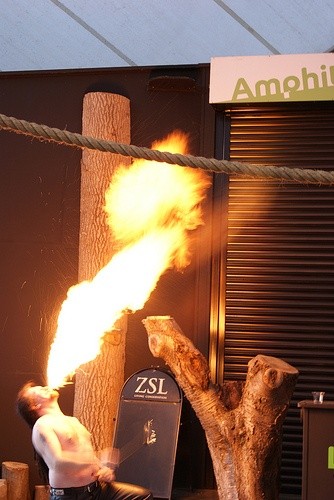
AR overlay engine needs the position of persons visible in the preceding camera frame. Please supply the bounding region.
[17,383,154,500]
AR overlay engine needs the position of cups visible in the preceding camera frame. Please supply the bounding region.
[312,391,325,404]
